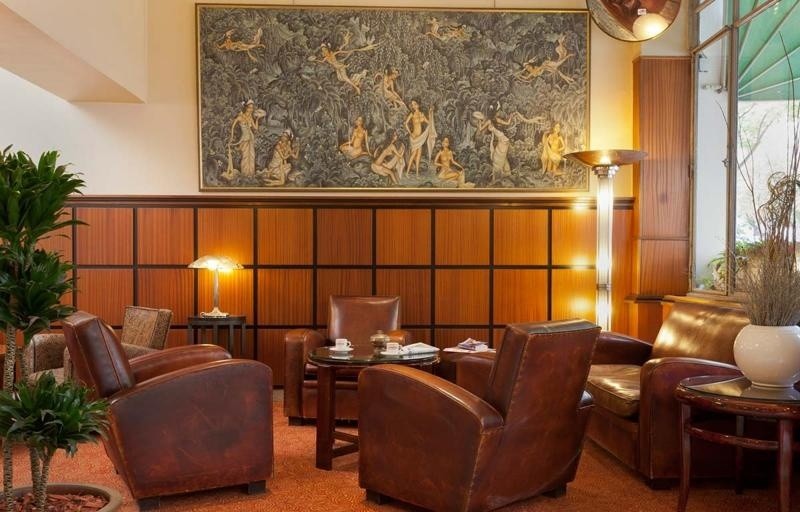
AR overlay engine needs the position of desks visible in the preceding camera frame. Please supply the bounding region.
[674,372,799,512]
[187,313,247,361]
[444,349,489,383]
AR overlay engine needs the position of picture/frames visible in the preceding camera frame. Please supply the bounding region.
[196,1,592,192]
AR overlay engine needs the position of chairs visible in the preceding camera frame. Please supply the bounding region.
[582,304,755,485]
[357,319,602,511]
[62,312,272,511]
[19,307,173,398]
[283,295,404,429]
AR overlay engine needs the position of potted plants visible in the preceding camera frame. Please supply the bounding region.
[0,143,121,511]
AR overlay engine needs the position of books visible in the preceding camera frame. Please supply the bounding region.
[458,336,488,351]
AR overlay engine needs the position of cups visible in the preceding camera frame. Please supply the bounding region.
[387,343,402,354]
[335,338,351,348]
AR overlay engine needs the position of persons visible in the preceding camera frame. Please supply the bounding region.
[514,54,577,79]
[232,98,262,172]
[267,127,300,179]
[339,115,372,160]
[372,68,408,107]
[318,41,363,94]
[368,128,405,187]
[477,100,515,179]
[401,97,435,175]
[434,134,466,181]
[545,122,565,171]
[215,35,267,53]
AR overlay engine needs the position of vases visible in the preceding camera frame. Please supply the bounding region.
[728,318,800,392]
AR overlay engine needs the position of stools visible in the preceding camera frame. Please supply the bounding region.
[1,343,18,389]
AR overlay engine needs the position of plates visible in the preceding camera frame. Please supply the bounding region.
[381,352,406,357]
[329,348,354,353]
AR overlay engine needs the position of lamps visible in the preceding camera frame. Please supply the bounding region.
[189,255,242,315]
[627,0,672,43]
[562,148,649,331]
